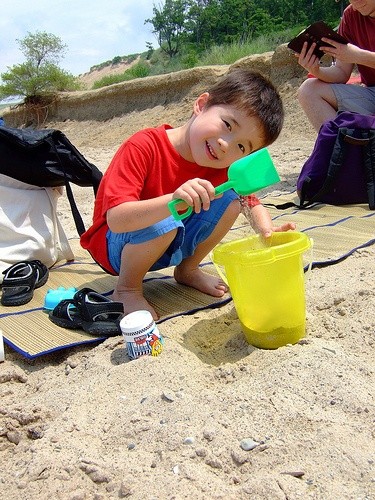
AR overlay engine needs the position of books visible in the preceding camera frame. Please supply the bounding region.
[286,21,349,60]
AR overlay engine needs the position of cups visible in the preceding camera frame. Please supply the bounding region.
[120,310,163,360]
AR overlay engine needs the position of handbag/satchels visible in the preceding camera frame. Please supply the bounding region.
[1,173,75,268]
[0,123,104,235]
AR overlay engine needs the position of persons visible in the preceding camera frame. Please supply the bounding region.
[80,68,297,320]
[296,0,375,134]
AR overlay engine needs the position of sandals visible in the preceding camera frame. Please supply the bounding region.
[1,257,51,308]
[47,286,125,337]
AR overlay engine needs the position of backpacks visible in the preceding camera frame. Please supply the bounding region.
[264,109,375,209]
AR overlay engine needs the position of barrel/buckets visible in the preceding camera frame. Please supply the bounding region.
[212,232,313,348]
